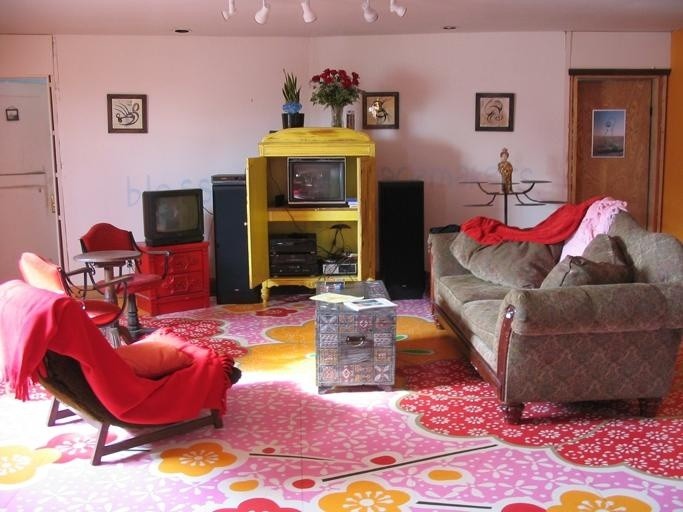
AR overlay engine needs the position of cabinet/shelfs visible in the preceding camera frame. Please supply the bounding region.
[246,125,377,309]
[130,240,210,317]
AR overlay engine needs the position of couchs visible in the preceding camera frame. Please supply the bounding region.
[425,208,683,424]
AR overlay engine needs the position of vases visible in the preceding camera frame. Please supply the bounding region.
[282,113,304,128]
[329,104,343,127]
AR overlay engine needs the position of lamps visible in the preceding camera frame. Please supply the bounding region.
[221,0,407,24]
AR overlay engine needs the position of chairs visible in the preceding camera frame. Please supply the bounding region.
[79,222,175,342]
[19,252,122,349]
[0,279,243,466]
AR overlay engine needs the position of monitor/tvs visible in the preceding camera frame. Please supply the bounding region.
[141,187,204,248]
[286,157,346,206]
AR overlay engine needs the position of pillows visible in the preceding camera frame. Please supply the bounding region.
[539,232,636,290]
[450,229,566,288]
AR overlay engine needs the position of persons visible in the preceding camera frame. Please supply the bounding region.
[498,148,514,193]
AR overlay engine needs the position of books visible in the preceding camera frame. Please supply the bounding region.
[343,297,398,312]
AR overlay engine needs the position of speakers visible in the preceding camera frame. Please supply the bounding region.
[377,180,425,303]
[212,185,262,305]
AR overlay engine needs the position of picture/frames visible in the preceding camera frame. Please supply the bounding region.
[107,93,148,133]
[362,92,399,130]
[474,92,515,131]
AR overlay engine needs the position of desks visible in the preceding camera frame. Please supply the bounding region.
[73,250,142,349]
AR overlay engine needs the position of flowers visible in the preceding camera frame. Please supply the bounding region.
[282,68,302,113]
[309,68,360,127]
